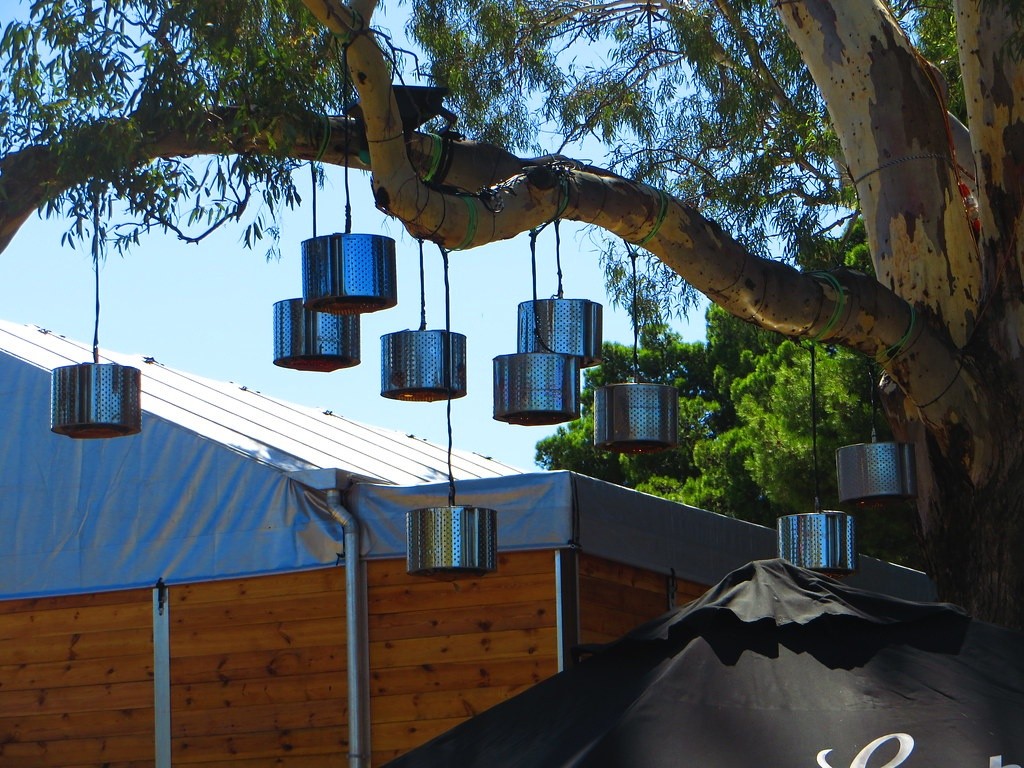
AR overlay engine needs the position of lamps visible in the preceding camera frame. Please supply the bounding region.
[517,218,602,368]
[405,248,498,578]
[301,44,397,314]
[594,252,679,454]
[835,356,917,507]
[50,176,142,439]
[777,344,857,576]
[339,85,463,140]
[493,228,581,426]
[381,239,467,402]
[274,159,360,371]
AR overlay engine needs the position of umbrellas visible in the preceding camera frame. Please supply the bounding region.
[377,557,1024,768]
